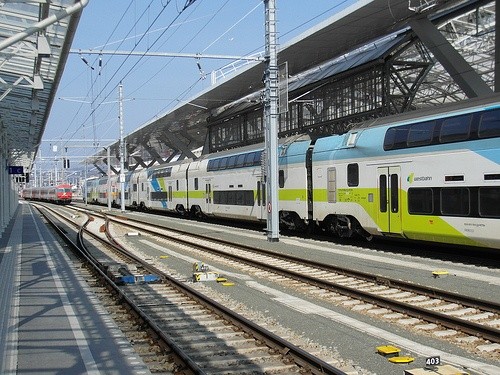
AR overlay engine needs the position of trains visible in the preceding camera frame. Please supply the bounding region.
[21,184,72,203]
[84,101,500,250]
